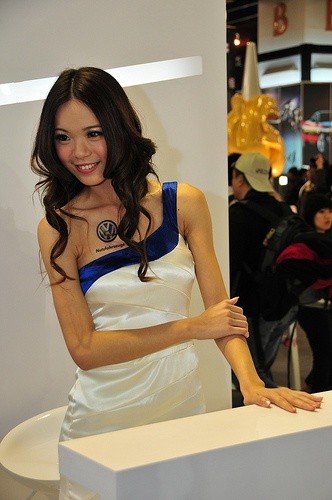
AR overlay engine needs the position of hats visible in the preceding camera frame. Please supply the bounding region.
[235,153,275,192]
[305,194,332,226]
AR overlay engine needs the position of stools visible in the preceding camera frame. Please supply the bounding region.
[0,403,76,499]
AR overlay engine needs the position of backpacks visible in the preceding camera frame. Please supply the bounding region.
[235,200,309,292]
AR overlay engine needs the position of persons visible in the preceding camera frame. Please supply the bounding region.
[227,145,332,409]
[27,66,323,500]
